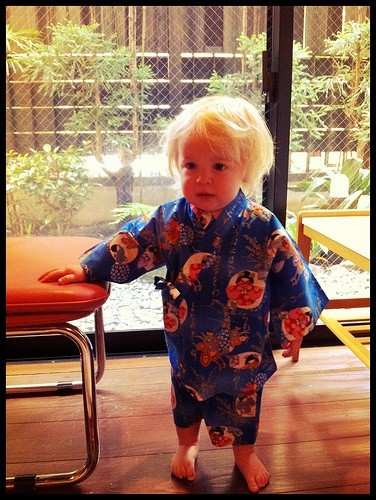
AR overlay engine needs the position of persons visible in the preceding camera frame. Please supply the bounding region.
[37,93,329,492]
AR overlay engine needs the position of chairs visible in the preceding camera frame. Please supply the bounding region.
[5,237,112,493]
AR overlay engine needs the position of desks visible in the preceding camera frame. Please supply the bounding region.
[290,208,371,373]
